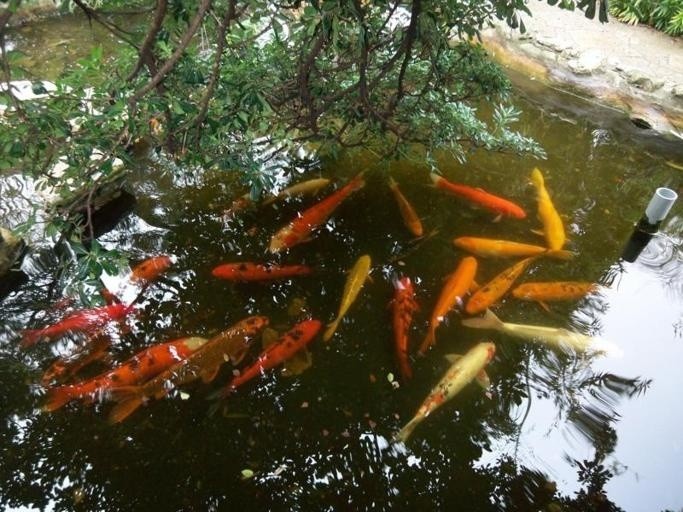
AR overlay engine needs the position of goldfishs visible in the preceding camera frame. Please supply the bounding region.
[19,254,322,425]
